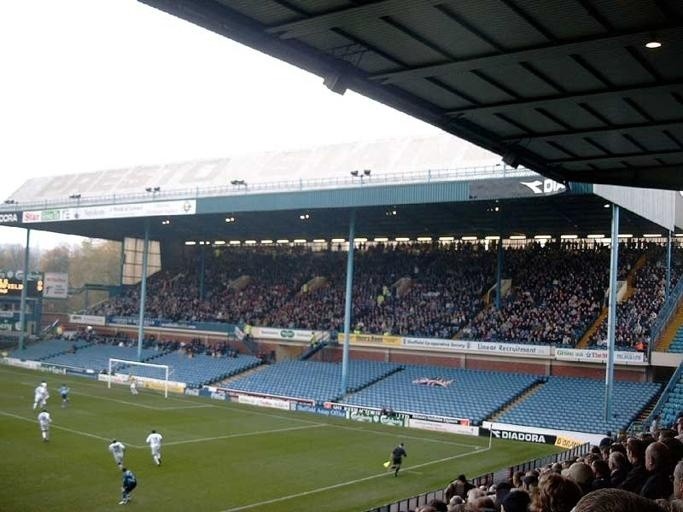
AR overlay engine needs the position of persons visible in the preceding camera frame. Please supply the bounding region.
[412,411,683,512]
[33,380,50,412]
[146,429,162,466]
[119,468,137,504]
[58,384,72,408]
[108,439,126,470]
[37,408,52,441]
[379,407,396,416]
[389,442,407,477]
[123,373,139,395]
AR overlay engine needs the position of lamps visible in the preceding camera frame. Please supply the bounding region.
[499,142,521,169]
[321,53,351,93]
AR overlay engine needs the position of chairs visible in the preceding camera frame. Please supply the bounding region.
[7,336,173,375]
[214,358,404,407]
[482,376,683,439]
[339,364,538,426]
[109,347,264,391]
